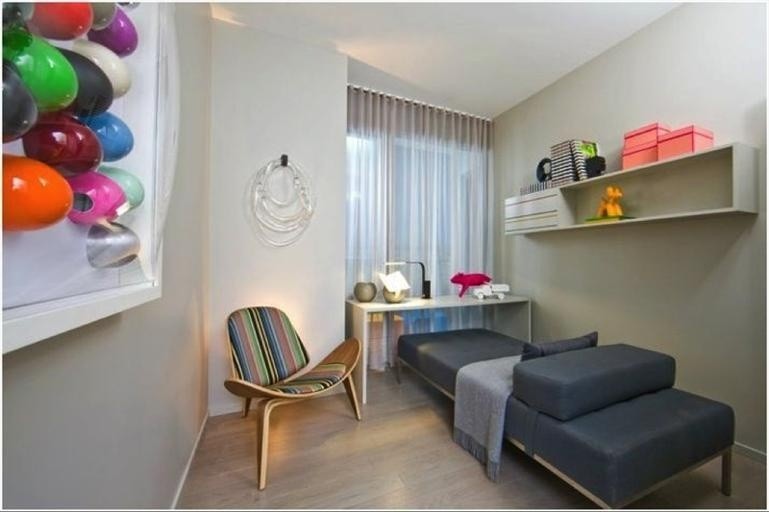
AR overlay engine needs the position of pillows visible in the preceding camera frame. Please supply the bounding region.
[522,330,599,363]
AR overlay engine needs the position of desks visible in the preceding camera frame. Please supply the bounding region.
[346,293,532,406]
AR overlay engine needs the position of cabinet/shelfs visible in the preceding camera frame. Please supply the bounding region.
[504,142,760,239]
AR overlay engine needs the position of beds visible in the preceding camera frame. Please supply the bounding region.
[395,327,735,510]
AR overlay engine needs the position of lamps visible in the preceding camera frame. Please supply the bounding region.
[385,260,431,298]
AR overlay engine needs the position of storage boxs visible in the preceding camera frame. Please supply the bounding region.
[656,125,714,161]
[623,122,673,149]
[621,140,657,170]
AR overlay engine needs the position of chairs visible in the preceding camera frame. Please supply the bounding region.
[222,305,364,492]
